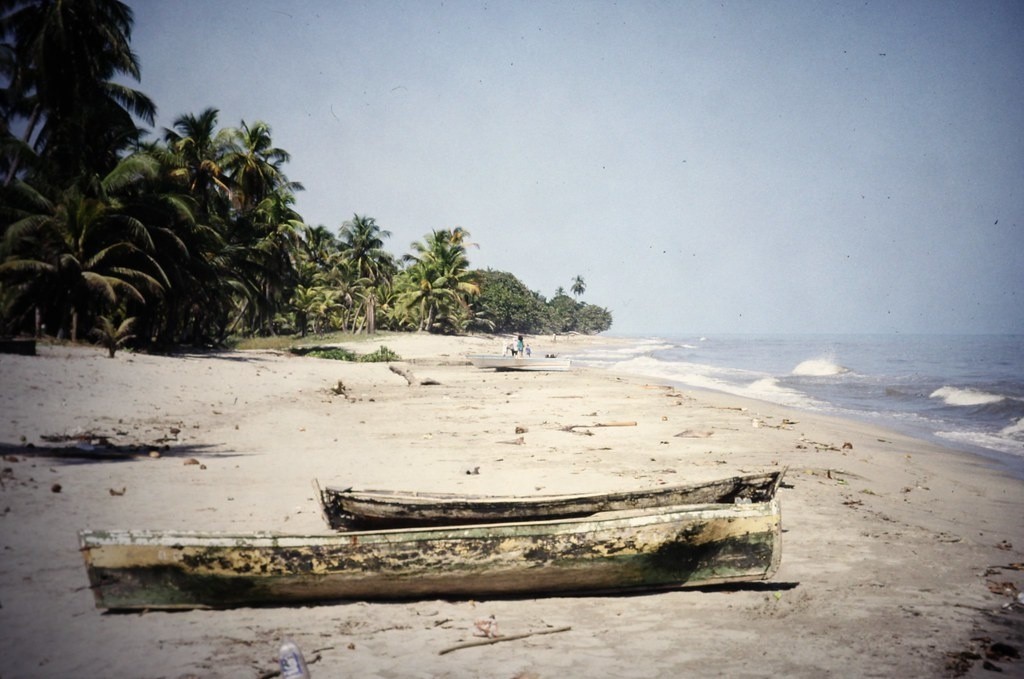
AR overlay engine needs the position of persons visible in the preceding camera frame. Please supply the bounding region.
[517,335,523,358]
[525,344,531,357]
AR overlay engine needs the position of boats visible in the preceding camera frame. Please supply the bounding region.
[318,462,792,530]
[77,502,785,611]
[465,352,571,374]
